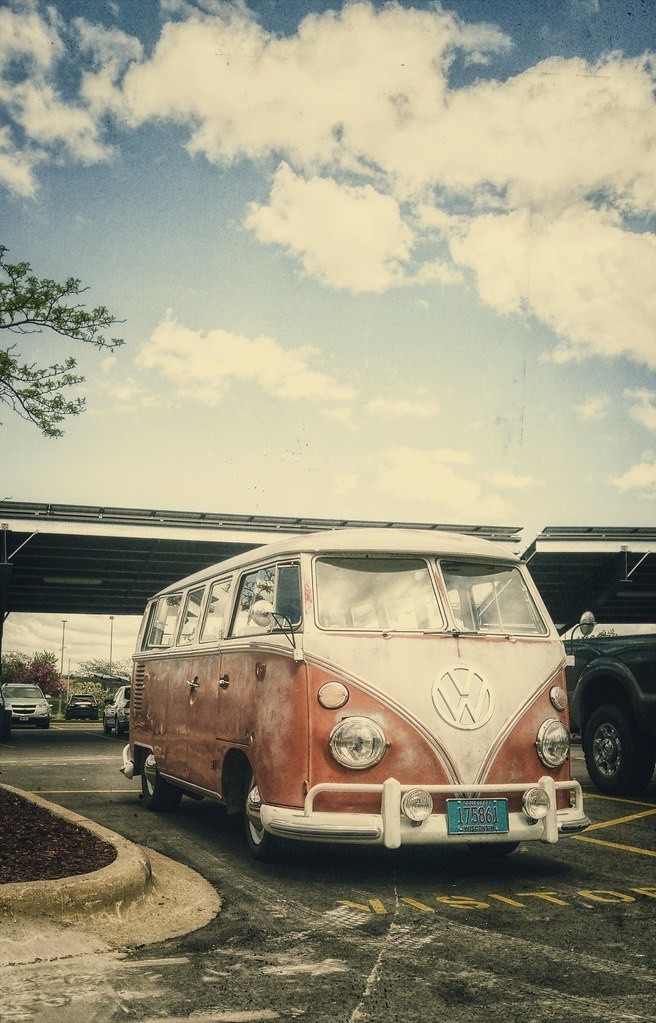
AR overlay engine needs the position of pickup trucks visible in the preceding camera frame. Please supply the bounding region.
[560,632,656,792]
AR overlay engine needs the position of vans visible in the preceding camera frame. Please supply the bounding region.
[121,526,595,864]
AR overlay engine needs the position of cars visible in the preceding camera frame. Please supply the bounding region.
[104,686,131,737]
[64,694,98,721]
[0,682,52,730]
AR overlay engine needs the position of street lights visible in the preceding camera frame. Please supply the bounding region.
[109,616,115,692]
[59,620,68,715]
